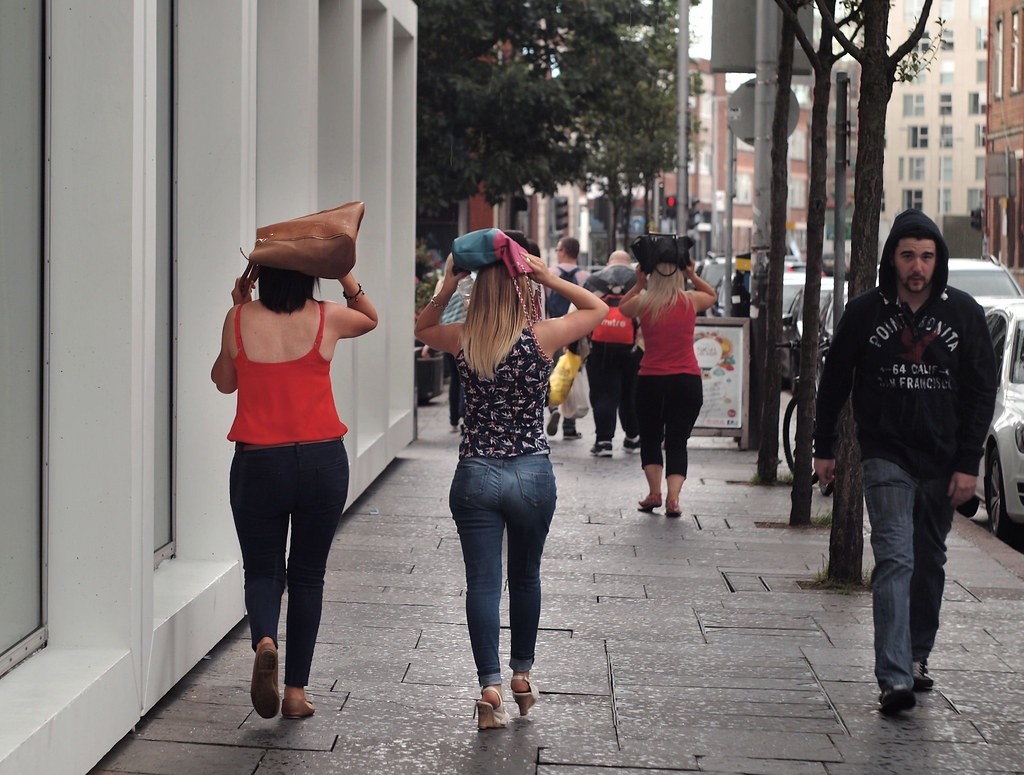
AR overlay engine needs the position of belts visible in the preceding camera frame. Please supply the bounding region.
[235,437,339,451]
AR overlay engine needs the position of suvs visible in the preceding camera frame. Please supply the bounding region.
[947,256,1023,300]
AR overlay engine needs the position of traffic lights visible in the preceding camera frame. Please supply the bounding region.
[554,196,568,232]
[665,196,675,219]
[971,210,981,231]
[688,199,699,230]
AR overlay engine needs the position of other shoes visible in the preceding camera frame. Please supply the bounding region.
[563,432,581,440]
[250,641,279,719]
[639,493,662,511]
[281,695,314,718]
[665,497,682,517]
[450,418,463,431]
[547,410,560,435]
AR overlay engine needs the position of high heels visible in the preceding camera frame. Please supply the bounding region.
[472,686,510,729]
[510,675,539,716]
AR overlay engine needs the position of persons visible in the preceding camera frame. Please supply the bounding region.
[415,255,610,729]
[563,249,647,457]
[434,236,589,442]
[618,255,718,517]
[813,208,997,711]
[210,271,378,720]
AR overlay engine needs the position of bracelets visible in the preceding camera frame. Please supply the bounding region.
[342,284,366,302]
[431,295,450,310]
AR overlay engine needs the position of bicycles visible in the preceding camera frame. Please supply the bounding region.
[773,335,837,495]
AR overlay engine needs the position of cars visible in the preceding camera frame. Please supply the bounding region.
[976,298,1024,548]
[785,239,826,278]
[822,240,852,280]
[782,273,849,390]
[702,256,736,317]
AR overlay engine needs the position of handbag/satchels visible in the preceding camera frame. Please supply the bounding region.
[453,228,552,365]
[239,202,365,296]
[545,349,582,401]
[630,231,695,277]
[559,362,589,419]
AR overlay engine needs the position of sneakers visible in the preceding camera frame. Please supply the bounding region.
[622,438,641,454]
[589,441,612,457]
[911,659,933,687]
[878,684,915,717]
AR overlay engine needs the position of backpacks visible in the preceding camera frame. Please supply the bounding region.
[586,273,639,349]
[547,262,581,318]
